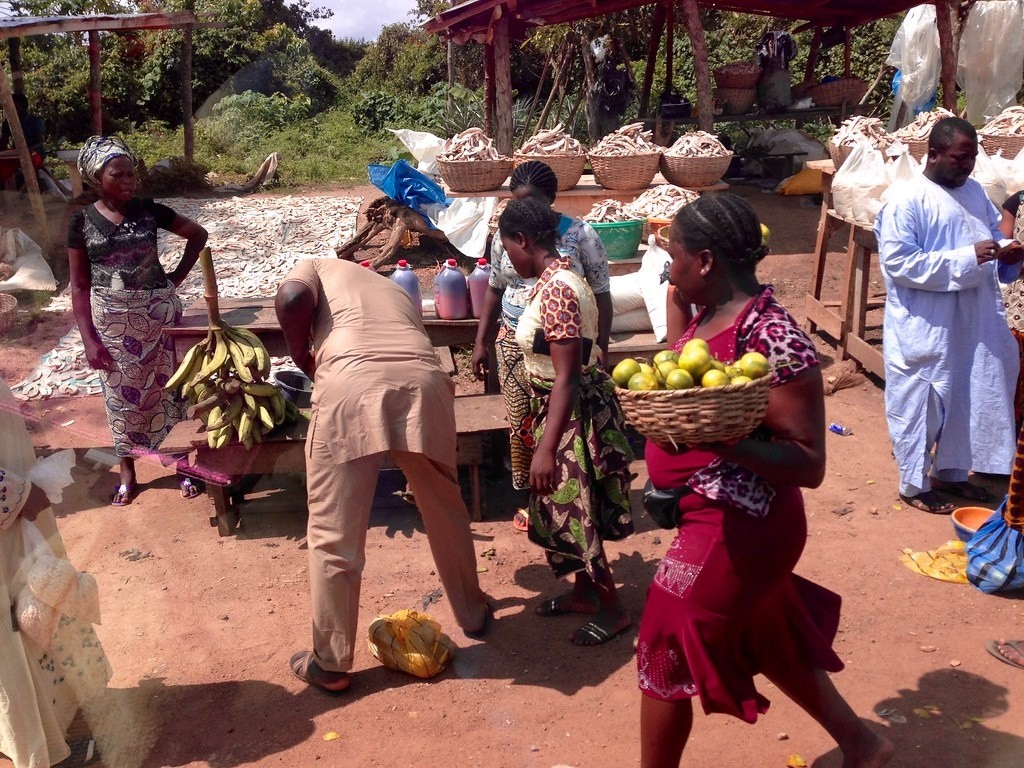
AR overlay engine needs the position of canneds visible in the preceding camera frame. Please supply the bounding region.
[829,422,851,435]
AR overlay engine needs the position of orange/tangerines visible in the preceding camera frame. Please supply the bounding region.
[612,338,767,391]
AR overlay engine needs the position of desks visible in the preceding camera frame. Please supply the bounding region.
[161,293,505,413]
[440,176,730,241]
[632,101,882,146]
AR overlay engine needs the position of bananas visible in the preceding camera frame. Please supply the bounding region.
[165,326,300,452]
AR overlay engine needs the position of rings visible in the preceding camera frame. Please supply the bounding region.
[990,248,996,255]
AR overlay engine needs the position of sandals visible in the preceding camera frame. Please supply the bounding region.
[899,490,959,515]
[929,476,999,503]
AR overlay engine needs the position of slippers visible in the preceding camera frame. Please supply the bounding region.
[569,614,632,646]
[986,639,1024,670]
[463,603,492,638]
[179,476,201,499]
[290,651,352,697]
[111,481,137,506]
[535,594,598,616]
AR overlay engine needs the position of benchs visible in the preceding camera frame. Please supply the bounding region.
[157,391,511,539]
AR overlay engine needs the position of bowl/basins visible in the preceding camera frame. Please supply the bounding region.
[588,220,644,261]
[273,370,314,408]
[951,506,996,543]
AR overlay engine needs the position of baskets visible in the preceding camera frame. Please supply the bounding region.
[0,293,17,335]
[614,357,772,450]
[513,151,587,192]
[713,88,757,115]
[892,138,928,163]
[588,149,662,190]
[790,78,870,106]
[829,137,891,172]
[642,216,673,242]
[713,68,763,88]
[435,153,514,191]
[979,133,1024,160]
[660,150,734,187]
[658,225,671,252]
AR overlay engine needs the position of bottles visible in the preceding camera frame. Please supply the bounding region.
[433,259,468,320]
[389,260,423,319]
[467,258,491,318]
[360,259,377,273]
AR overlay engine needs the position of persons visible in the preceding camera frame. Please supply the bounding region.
[471,161,613,531]
[66,135,208,506]
[0,93,45,199]
[635,192,894,768]
[498,197,634,648]
[871,117,1024,516]
[274,256,496,695]
[0,376,114,768]
